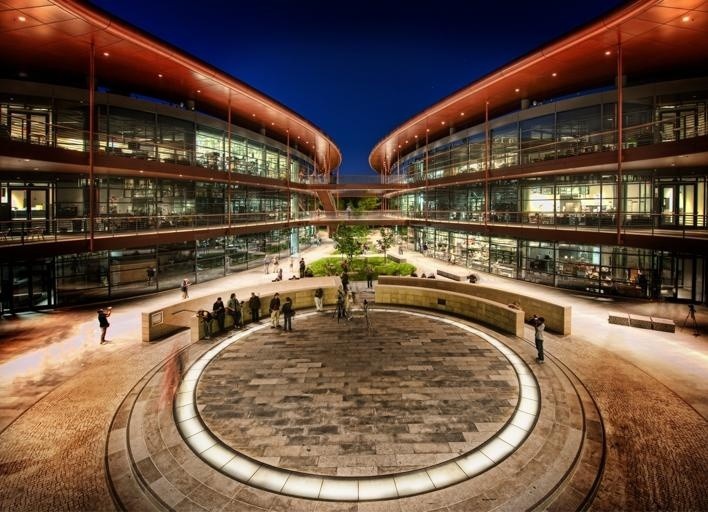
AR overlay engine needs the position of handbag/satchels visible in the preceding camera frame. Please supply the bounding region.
[228,310,236,315]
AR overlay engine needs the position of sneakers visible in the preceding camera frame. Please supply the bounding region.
[204,318,292,340]
[536,357,545,364]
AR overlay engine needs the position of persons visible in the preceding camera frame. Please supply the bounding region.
[423,242,428,256]
[264,255,349,330]
[421,274,425,278]
[147,266,155,286]
[196,311,213,340]
[365,264,374,288]
[227,294,241,328]
[427,273,434,278]
[535,317,545,363]
[96,308,112,345]
[467,274,477,283]
[411,270,417,276]
[249,292,260,322]
[181,279,190,299]
[213,298,225,330]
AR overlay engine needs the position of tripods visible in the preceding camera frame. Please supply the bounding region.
[682,308,697,329]
[333,299,347,324]
